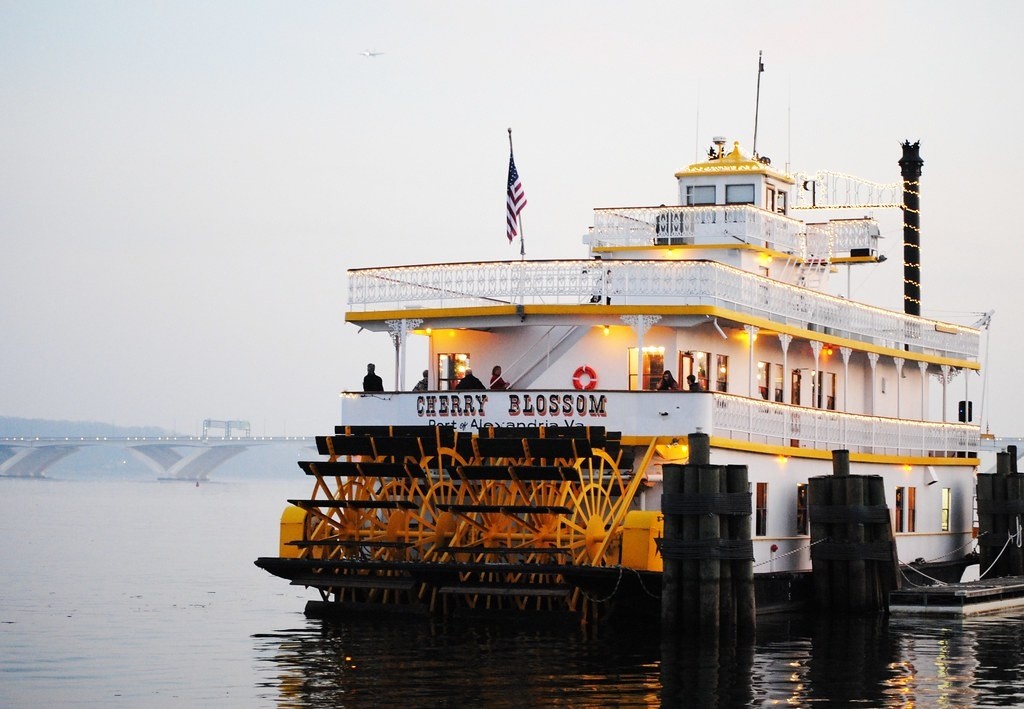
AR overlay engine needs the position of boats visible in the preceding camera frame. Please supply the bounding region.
[254,48,994,648]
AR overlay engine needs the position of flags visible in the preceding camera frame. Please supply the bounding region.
[506,154,528,244]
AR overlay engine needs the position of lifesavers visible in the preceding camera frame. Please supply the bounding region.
[572,365,598,391]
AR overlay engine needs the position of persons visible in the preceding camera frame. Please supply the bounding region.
[412,370,429,391]
[363,364,384,391]
[654,370,679,391]
[687,374,703,390]
[489,366,510,389]
[455,369,486,390]
